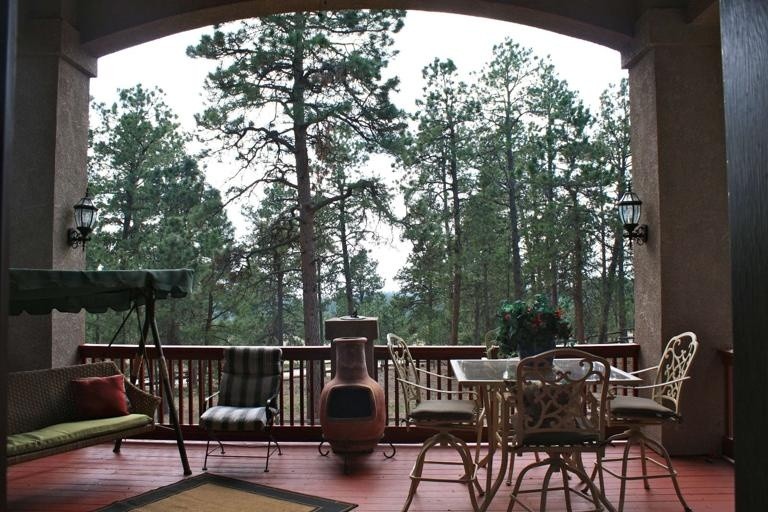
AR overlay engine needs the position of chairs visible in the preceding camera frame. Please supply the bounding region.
[583,330,701,512]
[508,346,610,512]
[386,332,488,512]
[480,325,575,486]
[199,346,283,472]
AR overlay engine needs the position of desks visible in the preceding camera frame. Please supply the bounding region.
[449,357,644,512]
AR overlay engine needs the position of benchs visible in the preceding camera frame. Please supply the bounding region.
[7,361,162,466]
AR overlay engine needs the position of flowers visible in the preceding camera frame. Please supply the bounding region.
[490,293,572,354]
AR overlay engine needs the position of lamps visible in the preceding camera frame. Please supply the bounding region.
[68,188,98,252]
[616,181,648,248]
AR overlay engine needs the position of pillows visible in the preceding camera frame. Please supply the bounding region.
[70,374,130,419]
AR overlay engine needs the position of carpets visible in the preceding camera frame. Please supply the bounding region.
[89,473,359,512]
